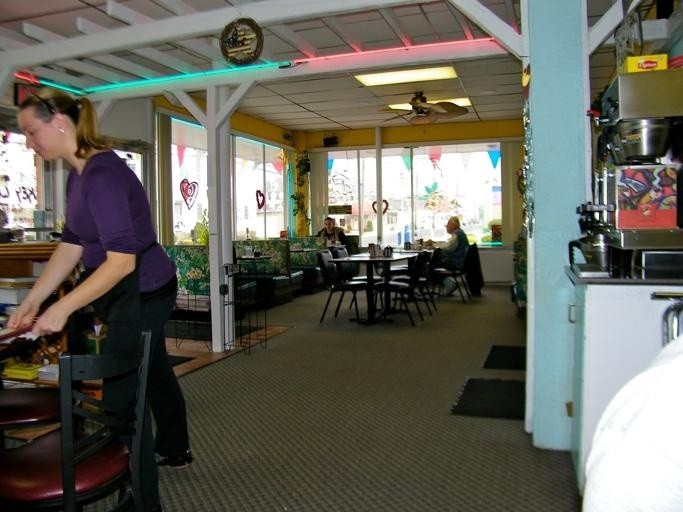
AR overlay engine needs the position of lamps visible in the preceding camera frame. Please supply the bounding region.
[407,115,430,125]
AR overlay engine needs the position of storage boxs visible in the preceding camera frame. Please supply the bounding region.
[0,276,40,305]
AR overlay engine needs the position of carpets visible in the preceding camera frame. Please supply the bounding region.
[450,376,525,420]
[483,344,526,370]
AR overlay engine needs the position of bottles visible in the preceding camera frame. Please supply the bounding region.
[403,239,433,250]
[367,244,393,257]
[245,245,261,257]
[34,204,45,228]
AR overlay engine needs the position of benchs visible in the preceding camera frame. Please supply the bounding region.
[160,235,360,323]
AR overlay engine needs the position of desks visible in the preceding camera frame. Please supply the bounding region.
[0,238,68,352]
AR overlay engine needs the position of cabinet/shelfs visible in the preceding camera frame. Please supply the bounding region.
[601,67,683,125]
[569,282,683,499]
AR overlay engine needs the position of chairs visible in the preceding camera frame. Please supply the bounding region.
[316,243,485,327]
[0,388,83,450]
[0,326,152,512]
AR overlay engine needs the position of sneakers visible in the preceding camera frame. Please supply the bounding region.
[443,280,461,297]
[130,447,193,471]
[107,493,163,512]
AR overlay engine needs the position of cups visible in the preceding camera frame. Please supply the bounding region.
[328,240,342,246]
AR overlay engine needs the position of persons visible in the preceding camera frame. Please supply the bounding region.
[6,86,193,511]
[426,216,469,296]
[315,217,348,246]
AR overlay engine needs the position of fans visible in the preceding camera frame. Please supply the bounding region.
[378,87,469,123]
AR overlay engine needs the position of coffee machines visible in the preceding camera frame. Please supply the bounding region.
[567,67,683,283]
